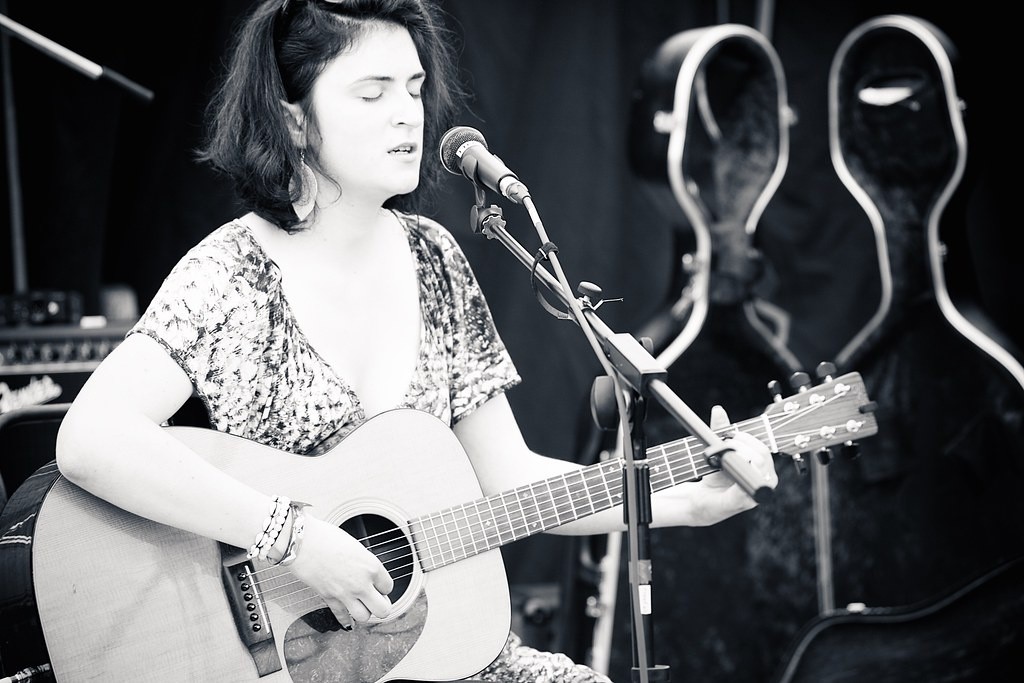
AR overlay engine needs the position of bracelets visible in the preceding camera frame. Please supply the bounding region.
[248,497,305,567]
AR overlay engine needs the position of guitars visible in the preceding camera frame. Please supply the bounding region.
[0,363,879,683]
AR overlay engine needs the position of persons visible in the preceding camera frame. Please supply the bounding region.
[56,0,777,683]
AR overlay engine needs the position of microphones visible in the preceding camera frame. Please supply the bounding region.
[439,126,530,206]
